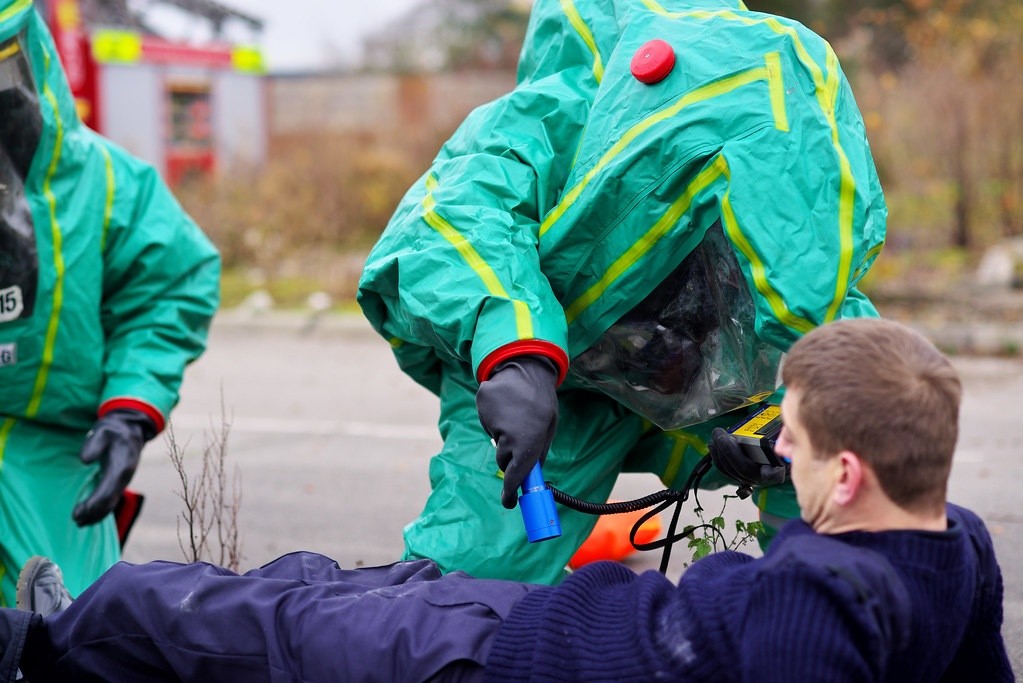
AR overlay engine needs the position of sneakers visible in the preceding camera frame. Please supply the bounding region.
[15,555,74,617]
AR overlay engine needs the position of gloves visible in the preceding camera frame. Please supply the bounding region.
[708,427,791,501]
[477,354,559,509]
[72,406,158,528]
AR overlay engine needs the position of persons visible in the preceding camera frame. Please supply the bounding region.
[0,316,1013,683]
[356,0,888,584]
[0,1,219,612]
